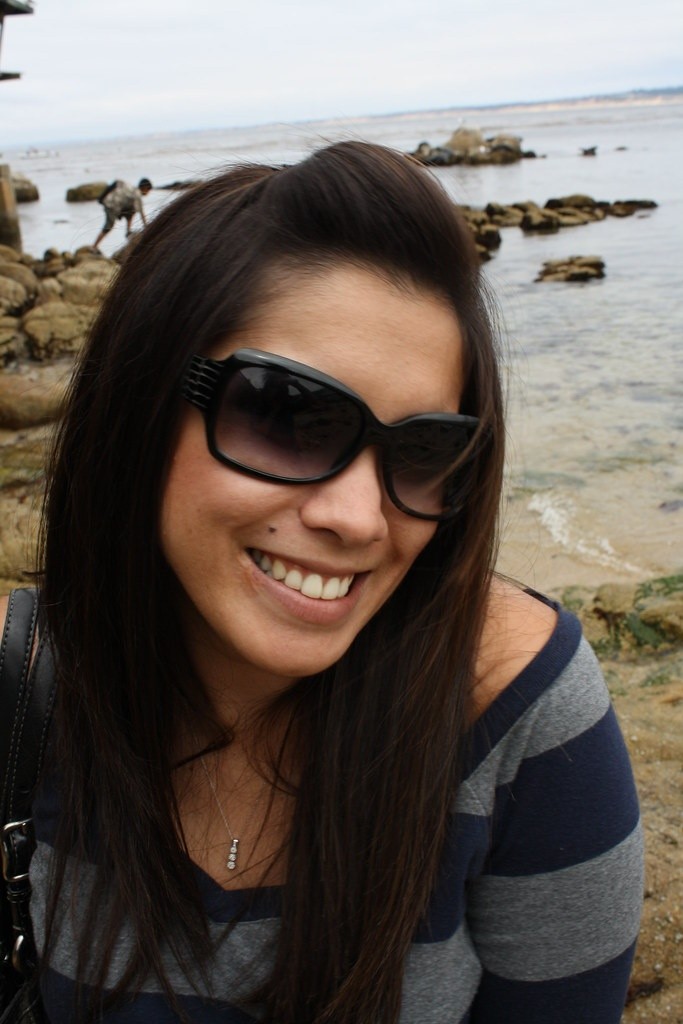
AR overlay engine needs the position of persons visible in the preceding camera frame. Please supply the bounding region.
[1,141,637,1022]
[87,175,153,249]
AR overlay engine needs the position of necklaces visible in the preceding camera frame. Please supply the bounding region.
[207,751,275,874]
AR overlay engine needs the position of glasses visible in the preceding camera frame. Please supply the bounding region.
[183,346,488,522]
